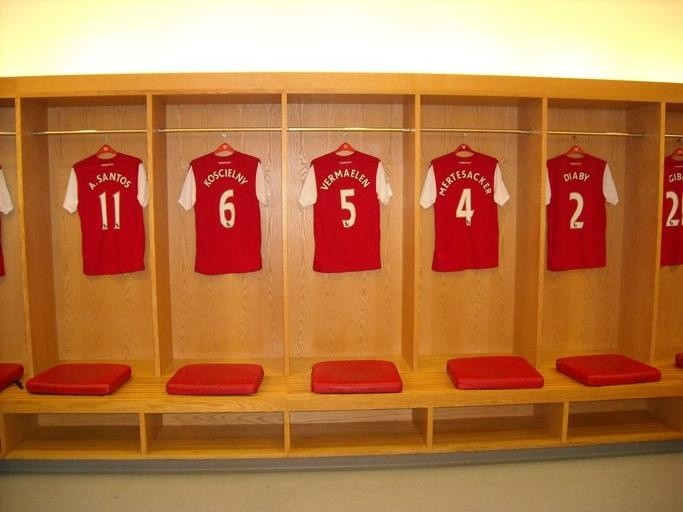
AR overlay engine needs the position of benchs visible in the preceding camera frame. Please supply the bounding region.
[0,359,682,473]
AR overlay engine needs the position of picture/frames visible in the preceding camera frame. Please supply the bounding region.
[454,130,473,156]
[335,129,354,155]
[566,134,583,159]
[92,130,119,159]
[671,138,682,161]
[213,131,235,156]
